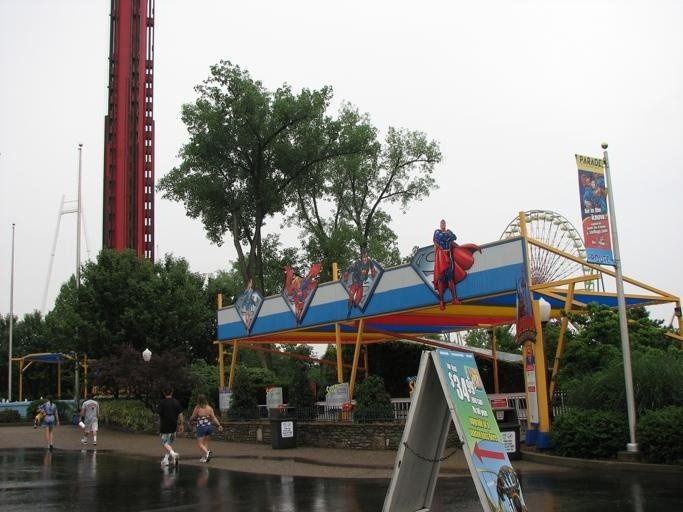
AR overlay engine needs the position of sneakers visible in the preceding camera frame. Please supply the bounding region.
[199,458,208,463]
[207,451,212,460]
[160,460,170,466]
[173,452,180,466]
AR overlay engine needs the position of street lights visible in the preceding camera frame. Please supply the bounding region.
[143,348,152,408]
[539,297,553,425]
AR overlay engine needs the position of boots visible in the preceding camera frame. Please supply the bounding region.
[448,280,464,305]
[353,298,361,309]
[438,285,445,312]
[346,303,352,321]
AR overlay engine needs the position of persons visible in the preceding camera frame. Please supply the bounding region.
[39,395,59,450]
[288,273,312,326]
[432,220,482,310]
[188,394,223,463]
[79,393,99,445]
[583,180,607,214]
[343,254,375,319]
[525,345,535,368]
[243,278,253,328]
[157,385,184,466]
